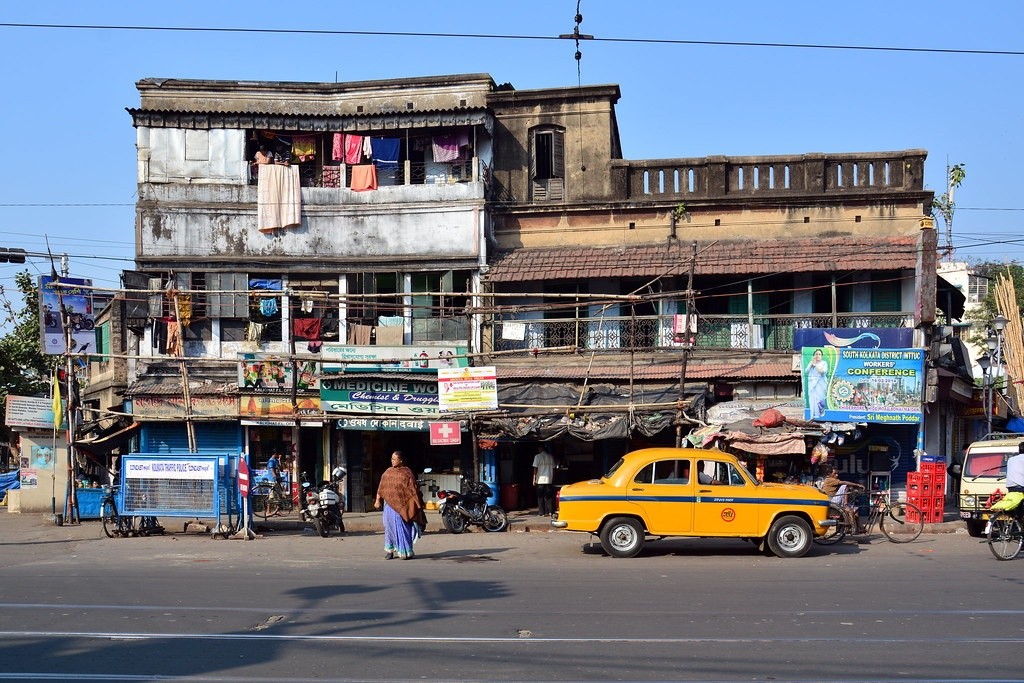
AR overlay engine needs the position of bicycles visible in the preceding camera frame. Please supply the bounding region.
[247,471,316,518]
[98,484,158,537]
[979,510,1024,561]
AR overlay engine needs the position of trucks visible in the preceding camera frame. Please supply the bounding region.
[954,433,1024,537]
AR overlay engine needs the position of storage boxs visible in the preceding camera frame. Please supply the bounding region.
[319,492,335,505]
[906,455,946,524]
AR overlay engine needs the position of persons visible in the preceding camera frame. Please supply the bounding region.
[252,144,273,165]
[373,450,428,560]
[698,460,727,484]
[805,348,828,419]
[1006,441,1024,518]
[266,448,282,516]
[822,465,865,521]
[532,443,553,517]
[274,145,290,166]
[955,444,970,466]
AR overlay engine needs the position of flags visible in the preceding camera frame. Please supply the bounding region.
[51,370,63,431]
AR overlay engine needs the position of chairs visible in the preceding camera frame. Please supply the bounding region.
[678,464,690,479]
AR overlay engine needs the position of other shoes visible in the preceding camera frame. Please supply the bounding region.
[275,512,286,517]
[384,553,393,560]
[265,513,276,517]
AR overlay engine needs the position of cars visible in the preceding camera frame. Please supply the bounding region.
[549,448,830,559]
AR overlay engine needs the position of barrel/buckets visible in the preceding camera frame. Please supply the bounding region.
[501,483,518,511]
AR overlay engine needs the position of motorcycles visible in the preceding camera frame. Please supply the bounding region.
[435,475,507,533]
[415,468,433,532]
[71,314,94,331]
[301,467,350,537]
[45,310,57,327]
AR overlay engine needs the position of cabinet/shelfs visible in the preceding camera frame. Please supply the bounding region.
[869,471,891,516]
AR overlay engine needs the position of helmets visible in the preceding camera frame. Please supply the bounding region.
[333,467,347,479]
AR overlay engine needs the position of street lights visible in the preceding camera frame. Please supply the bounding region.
[976,314,1011,440]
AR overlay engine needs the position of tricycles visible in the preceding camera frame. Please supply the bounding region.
[812,488,924,545]
